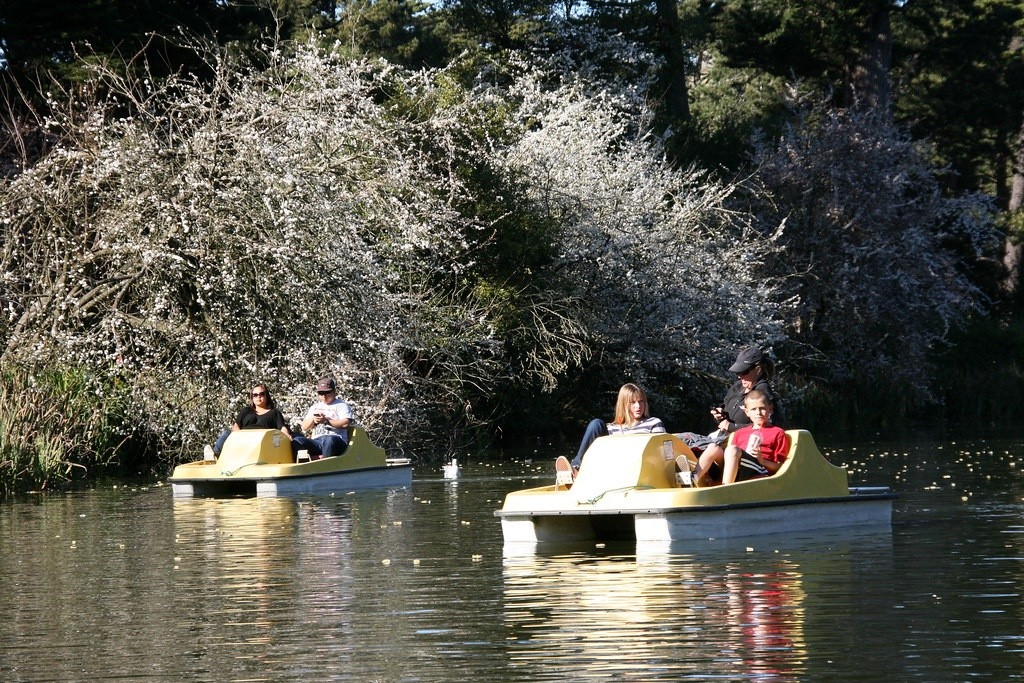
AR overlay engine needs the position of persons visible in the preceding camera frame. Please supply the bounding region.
[203,383,293,461]
[290,378,355,463]
[555,383,666,491]
[671,346,782,458]
[675,389,790,488]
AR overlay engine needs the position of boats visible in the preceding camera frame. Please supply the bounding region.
[165,424,413,500]
[490,427,895,542]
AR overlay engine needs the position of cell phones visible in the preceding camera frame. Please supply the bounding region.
[313,413,321,417]
[711,407,725,417]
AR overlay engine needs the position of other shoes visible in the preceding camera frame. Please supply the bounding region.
[204,444,214,460]
[675,455,697,488]
[555,455,575,489]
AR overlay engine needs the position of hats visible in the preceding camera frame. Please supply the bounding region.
[317,379,334,391]
[728,347,763,373]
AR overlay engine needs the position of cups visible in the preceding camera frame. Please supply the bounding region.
[745,434,761,455]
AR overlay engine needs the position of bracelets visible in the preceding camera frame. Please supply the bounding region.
[324,419,328,424]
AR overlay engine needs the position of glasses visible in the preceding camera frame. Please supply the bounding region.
[734,362,758,375]
[318,391,331,395]
[252,392,266,398]
